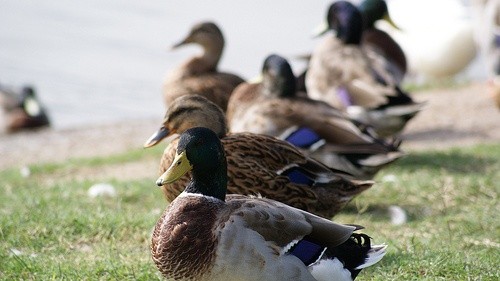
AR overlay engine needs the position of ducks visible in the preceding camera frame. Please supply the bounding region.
[293,0,426,147]
[142,95,375,222]
[159,22,246,114]
[147,127,387,280]
[226,57,404,175]
[0,85,49,132]
